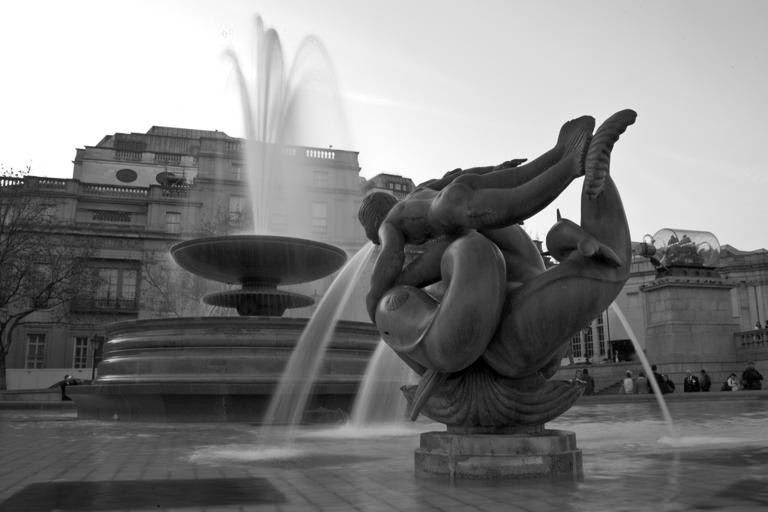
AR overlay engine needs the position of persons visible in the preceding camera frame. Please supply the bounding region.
[357,115,597,325]
[742,361,763,390]
[700,369,710,392]
[647,365,664,394]
[61,374,77,402]
[728,373,740,391]
[663,374,675,393]
[580,369,595,395]
[619,369,636,395]
[667,234,691,246]
[635,372,650,394]
[754,319,768,344]
[613,350,623,363]
[570,370,582,383]
[684,370,700,392]
